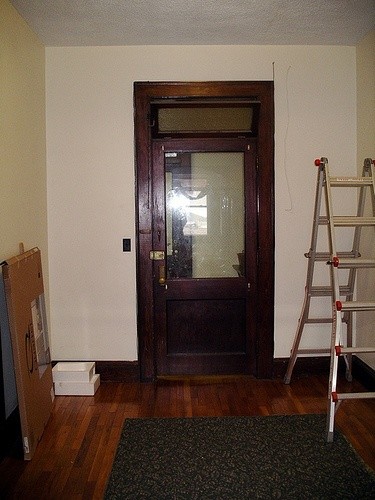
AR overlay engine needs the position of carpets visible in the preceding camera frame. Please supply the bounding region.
[100,413,375,500]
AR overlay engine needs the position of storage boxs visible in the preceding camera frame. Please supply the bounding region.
[51,361,96,382]
[53,374,101,396]
[0,242,56,463]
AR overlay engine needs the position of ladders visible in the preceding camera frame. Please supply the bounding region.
[284,157,374,443]
